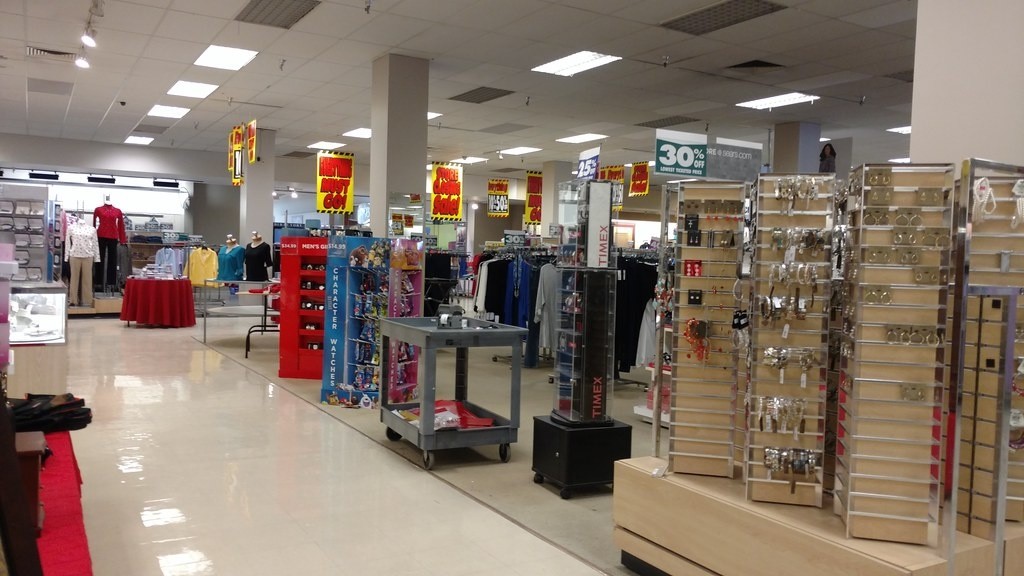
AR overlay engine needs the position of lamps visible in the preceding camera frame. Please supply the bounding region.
[75,0,105,68]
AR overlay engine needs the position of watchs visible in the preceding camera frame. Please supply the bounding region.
[301,265,326,351]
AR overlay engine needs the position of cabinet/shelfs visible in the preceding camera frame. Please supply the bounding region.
[203,279,280,342]
[278,236,421,409]
[0,178,69,404]
[16,431,45,539]
[124,230,164,269]
[613,156,1023,576]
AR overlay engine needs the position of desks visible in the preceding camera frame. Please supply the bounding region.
[234,292,280,359]
[121,277,196,328]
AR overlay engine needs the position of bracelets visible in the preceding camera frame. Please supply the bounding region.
[729,175,1024,481]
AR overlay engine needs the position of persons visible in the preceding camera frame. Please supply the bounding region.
[64,201,127,307]
[215,236,273,280]
[819,143,837,172]
